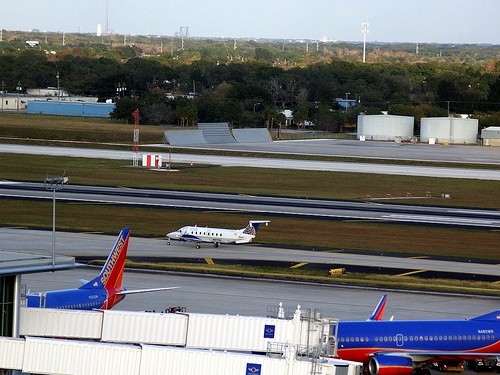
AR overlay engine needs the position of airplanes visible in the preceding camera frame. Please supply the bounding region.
[21,228,181,311]
[327,294,500,375]
[166,220,272,249]
